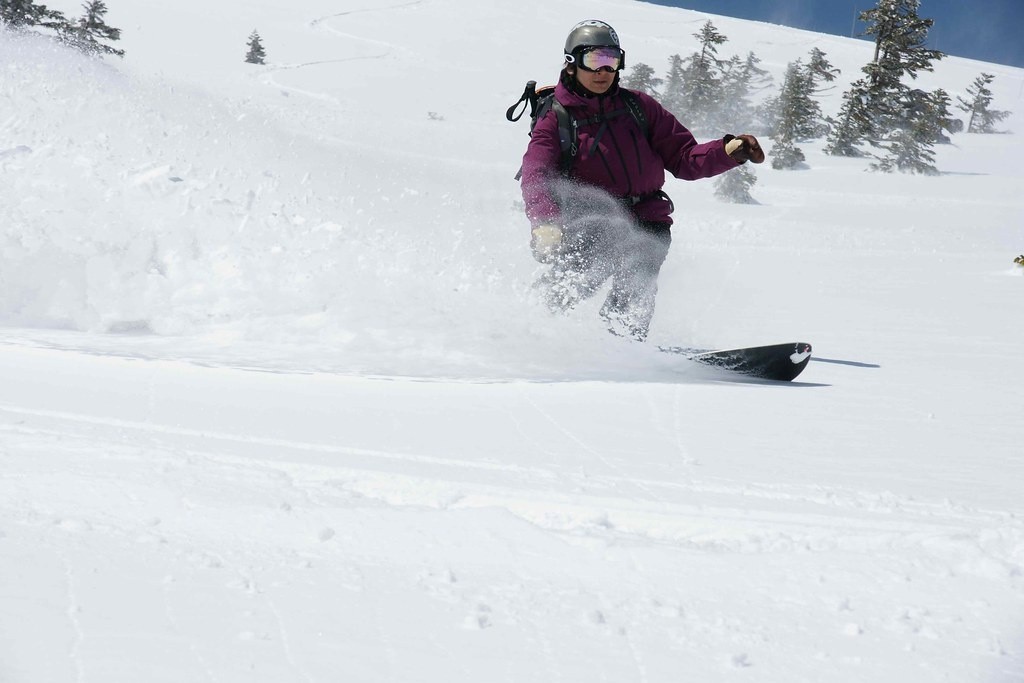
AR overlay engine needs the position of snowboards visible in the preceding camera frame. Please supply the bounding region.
[681,338,813,382]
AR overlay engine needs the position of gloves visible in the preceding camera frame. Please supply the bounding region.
[530,224,565,263]
[725,133,765,165]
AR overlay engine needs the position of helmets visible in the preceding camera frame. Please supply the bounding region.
[564,20,620,75]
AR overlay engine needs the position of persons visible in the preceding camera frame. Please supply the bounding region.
[523,19,764,343]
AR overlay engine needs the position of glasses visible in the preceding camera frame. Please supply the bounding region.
[575,45,625,72]
